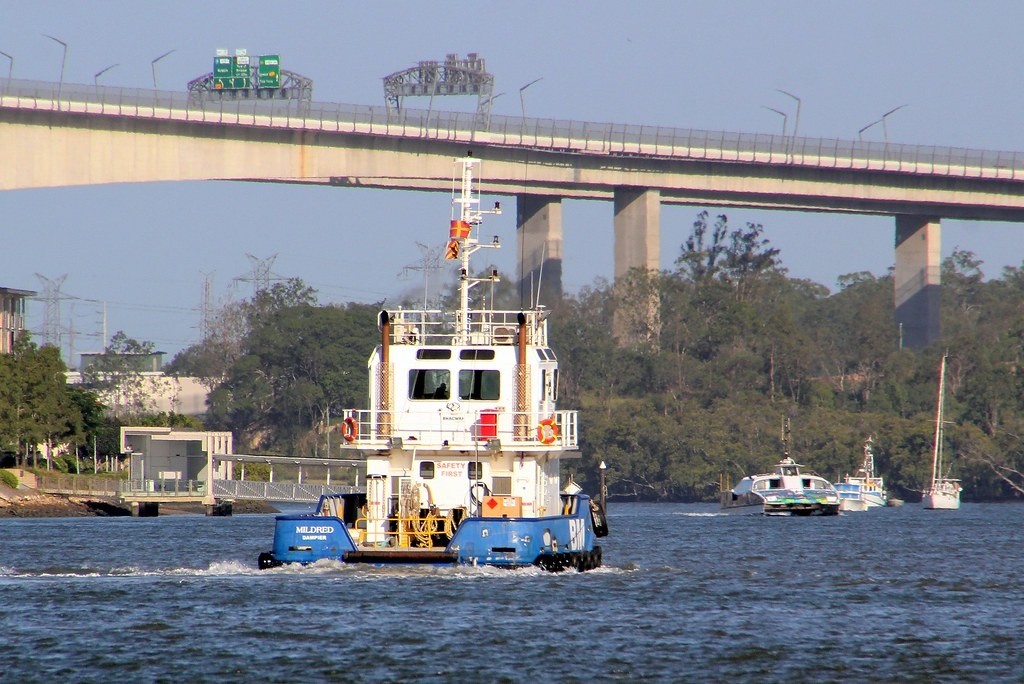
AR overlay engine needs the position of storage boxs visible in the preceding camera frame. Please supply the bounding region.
[564,481,582,494]
[482,496,522,517]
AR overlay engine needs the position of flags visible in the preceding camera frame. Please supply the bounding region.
[445,239,459,259]
[450,220,471,238]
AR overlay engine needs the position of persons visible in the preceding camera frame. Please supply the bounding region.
[434,383,447,399]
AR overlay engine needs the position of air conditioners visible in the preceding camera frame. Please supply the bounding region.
[492,327,519,345]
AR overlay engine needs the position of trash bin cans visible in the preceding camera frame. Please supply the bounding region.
[480,409,497,441]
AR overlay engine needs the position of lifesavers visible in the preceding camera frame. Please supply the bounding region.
[537,419,559,445]
[342,417,357,441]
[534,544,603,572]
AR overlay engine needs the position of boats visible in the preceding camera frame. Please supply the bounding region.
[729,456,840,506]
[889,498,904,507]
[762,499,840,516]
[257,150,609,575]
[832,434,887,506]
[838,499,869,512]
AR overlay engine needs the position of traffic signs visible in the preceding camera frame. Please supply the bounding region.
[213,56,251,90]
[257,54,280,89]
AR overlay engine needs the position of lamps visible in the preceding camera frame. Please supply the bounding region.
[391,438,402,447]
[491,439,500,447]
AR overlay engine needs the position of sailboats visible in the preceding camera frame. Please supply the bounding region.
[921,356,964,509]
